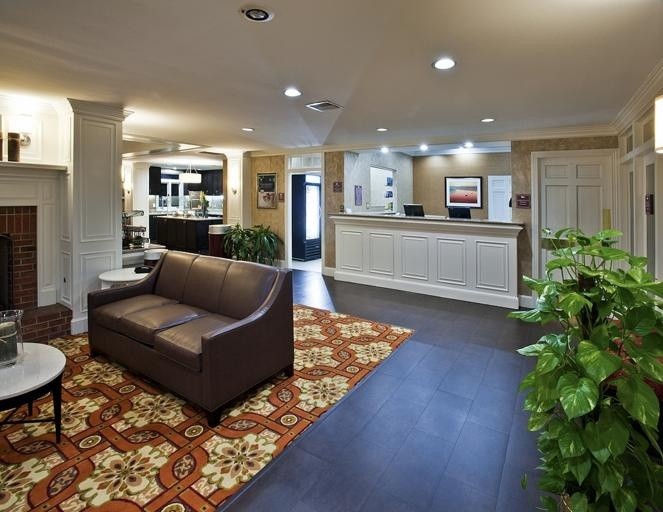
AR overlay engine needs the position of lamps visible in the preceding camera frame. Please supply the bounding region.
[178,158,202,184]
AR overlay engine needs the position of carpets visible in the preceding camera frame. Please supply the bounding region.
[0,303,416,512]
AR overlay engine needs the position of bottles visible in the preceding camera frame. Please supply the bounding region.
[183,210,188,218]
[172,211,177,217]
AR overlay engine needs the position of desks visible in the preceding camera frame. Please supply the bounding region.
[98,267,150,290]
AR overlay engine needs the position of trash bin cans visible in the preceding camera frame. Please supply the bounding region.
[208,225,232,259]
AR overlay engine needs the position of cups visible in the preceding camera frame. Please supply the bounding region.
[0,309,25,367]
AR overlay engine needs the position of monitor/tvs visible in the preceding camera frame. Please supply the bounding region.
[448,206,471,219]
[403,204,425,216]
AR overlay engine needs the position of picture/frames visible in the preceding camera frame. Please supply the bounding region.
[444,176,484,209]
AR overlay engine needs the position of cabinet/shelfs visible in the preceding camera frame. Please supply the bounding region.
[183,170,222,195]
[149,214,222,253]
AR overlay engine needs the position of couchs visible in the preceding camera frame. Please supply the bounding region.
[88,250,294,428]
